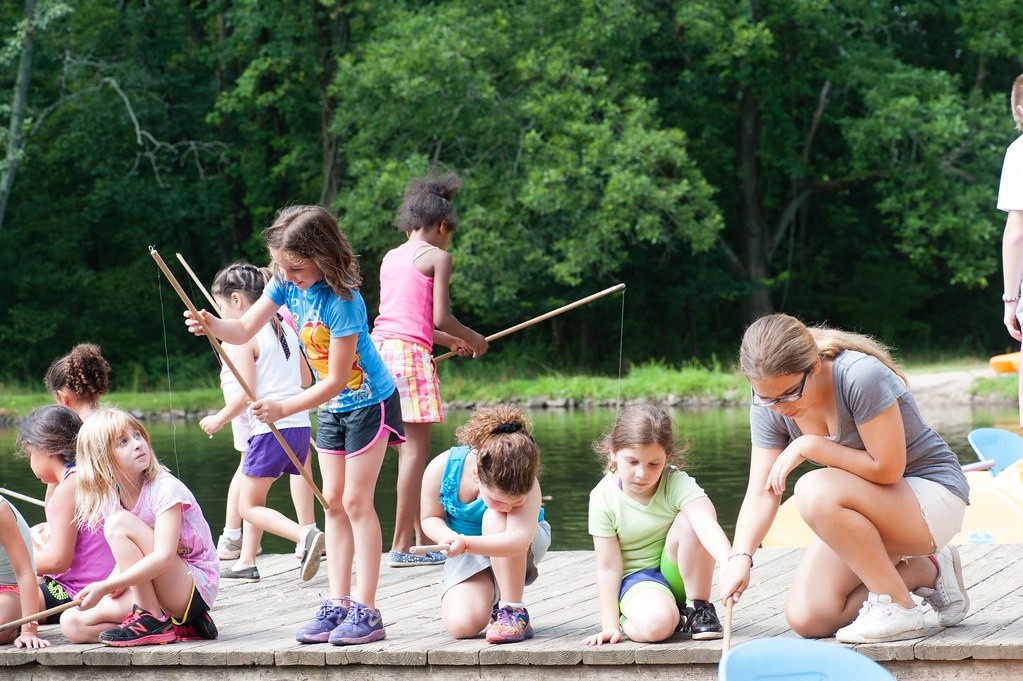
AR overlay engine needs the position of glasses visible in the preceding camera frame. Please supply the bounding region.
[752,369,810,407]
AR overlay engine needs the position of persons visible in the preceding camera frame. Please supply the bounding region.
[580,404,732,646]
[183,205,406,646]
[0,495,52,649]
[996,74,1023,440]
[718,314,971,643]
[369,173,488,569]
[58,406,220,648]
[198,261,325,583]
[12,343,118,625]
[419,404,552,645]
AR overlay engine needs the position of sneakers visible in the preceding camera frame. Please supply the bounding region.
[99,604,177,646]
[328,603,386,644]
[683,599,723,640]
[295,544,326,558]
[836,592,925,643]
[219,563,260,582]
[913,543,970,626]
[486,599,534,643]
[216,534,262,560]
[389,550,446,566]
[301,529,325,581]
[173,611,218,641]
[296,601,349,642]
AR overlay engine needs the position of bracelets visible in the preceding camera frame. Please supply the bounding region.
[728,552,754,568]
[1002,293,1020,302]
[460,534,469,554]
[23,620,39,627]
[459,534,469,554]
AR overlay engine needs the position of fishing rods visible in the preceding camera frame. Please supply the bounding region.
[434,281,626,364]
[0,486,45,507]
[174,252,222,318]
[720,595,734,656]
[0,599,83,633]
[147,243,332,513]
